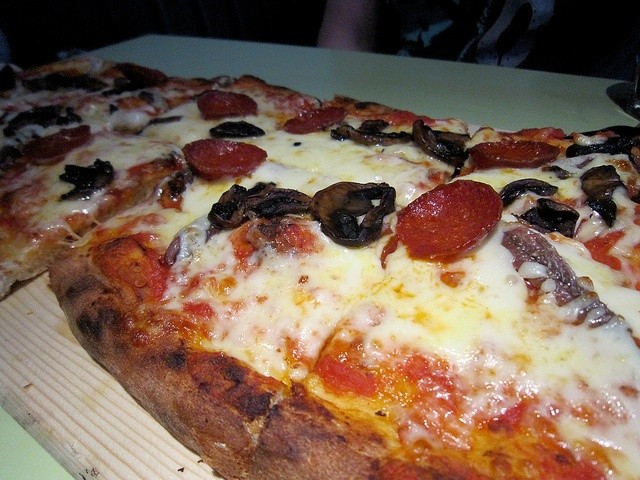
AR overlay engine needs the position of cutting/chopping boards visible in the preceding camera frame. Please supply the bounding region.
[1,273,218,480]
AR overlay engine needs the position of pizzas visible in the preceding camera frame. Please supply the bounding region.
[1,56,639,476]
